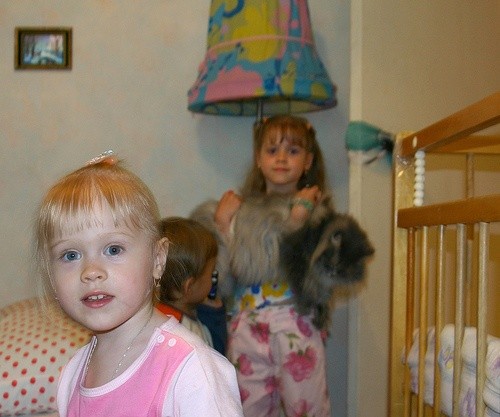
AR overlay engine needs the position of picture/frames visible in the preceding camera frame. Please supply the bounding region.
[13,25,73,72]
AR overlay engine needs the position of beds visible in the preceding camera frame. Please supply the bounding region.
[385,89,500,416]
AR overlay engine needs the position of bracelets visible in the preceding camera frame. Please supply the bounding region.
[291,196,313,211]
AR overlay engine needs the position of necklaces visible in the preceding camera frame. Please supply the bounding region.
[83,306,153,388]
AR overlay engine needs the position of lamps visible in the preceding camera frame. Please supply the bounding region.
[188,0,338,125]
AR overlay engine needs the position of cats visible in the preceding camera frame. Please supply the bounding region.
[188,186,377,332]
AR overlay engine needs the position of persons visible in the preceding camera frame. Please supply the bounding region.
[33,149,244,417]
[153,113,331,417]
